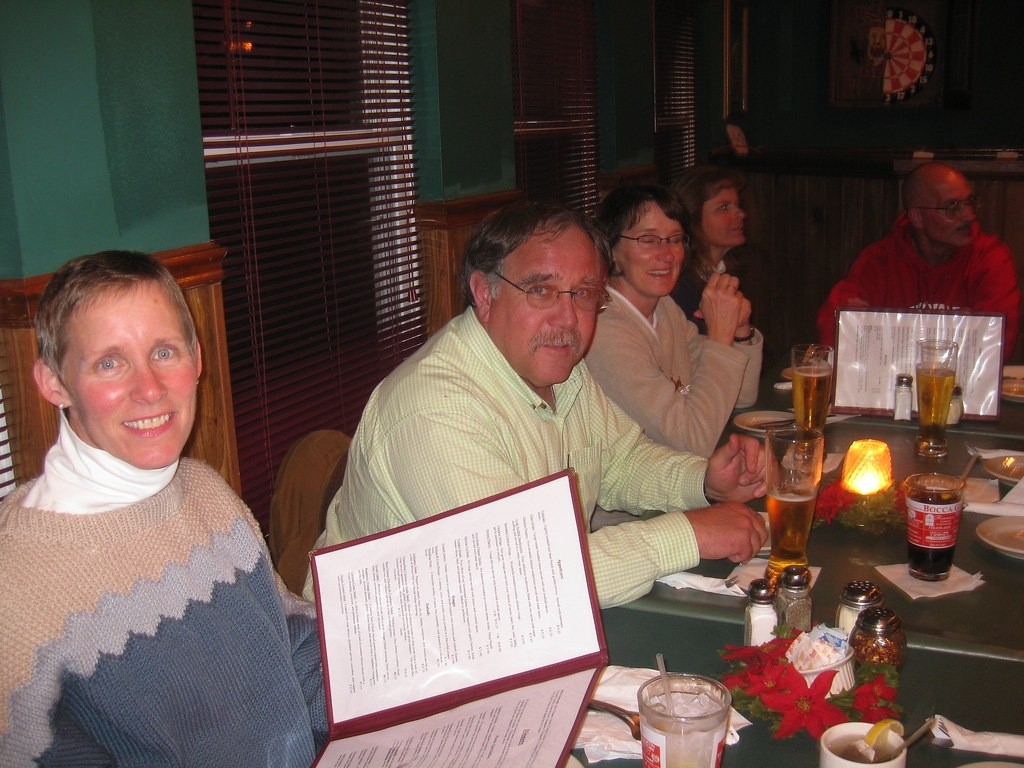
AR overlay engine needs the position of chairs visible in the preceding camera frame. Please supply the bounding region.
[272,430,353,591]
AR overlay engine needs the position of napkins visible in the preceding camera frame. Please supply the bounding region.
[926,713,1024,758]
[654,572,749,600]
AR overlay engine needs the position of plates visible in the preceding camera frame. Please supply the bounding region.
[975,516,1024,560]
[733,411,795,438]
[1002,365,1024,403]
[983,455,1024,486]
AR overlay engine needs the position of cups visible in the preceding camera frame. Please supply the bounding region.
[791,344,835,431]
[904,471,966,582]
[765,425,824,582]
[819,721,907,768]
[914,339,958,459]
[637,673,732,768]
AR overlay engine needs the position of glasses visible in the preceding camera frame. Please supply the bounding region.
[618,232,690,249]
[486,269,610,311]
[914,195,980,218]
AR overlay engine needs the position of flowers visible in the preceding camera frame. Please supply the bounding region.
[710,621,905,743]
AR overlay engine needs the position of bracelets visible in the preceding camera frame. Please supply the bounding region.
[734,327,758,342]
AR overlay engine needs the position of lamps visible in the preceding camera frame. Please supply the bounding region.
[842,439,893,498]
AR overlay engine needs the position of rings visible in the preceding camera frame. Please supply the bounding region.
[728,284,737,291]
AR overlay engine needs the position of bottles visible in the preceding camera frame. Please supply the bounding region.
[836,579,884,641]
[945,385,963,427]
[849,607,907,671]
[775,565,812,634]
[745,578,781,647]
[893,374,913,423]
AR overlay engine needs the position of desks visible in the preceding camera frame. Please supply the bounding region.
[566,368,1023,768]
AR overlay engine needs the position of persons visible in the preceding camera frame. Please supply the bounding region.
[302,199,777,615]
[663,165,773,399]
[817,162,1021,365]
[0,249,328,768]
[578,184,764,460]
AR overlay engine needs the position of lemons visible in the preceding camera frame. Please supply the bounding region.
[864,718,905,750]
